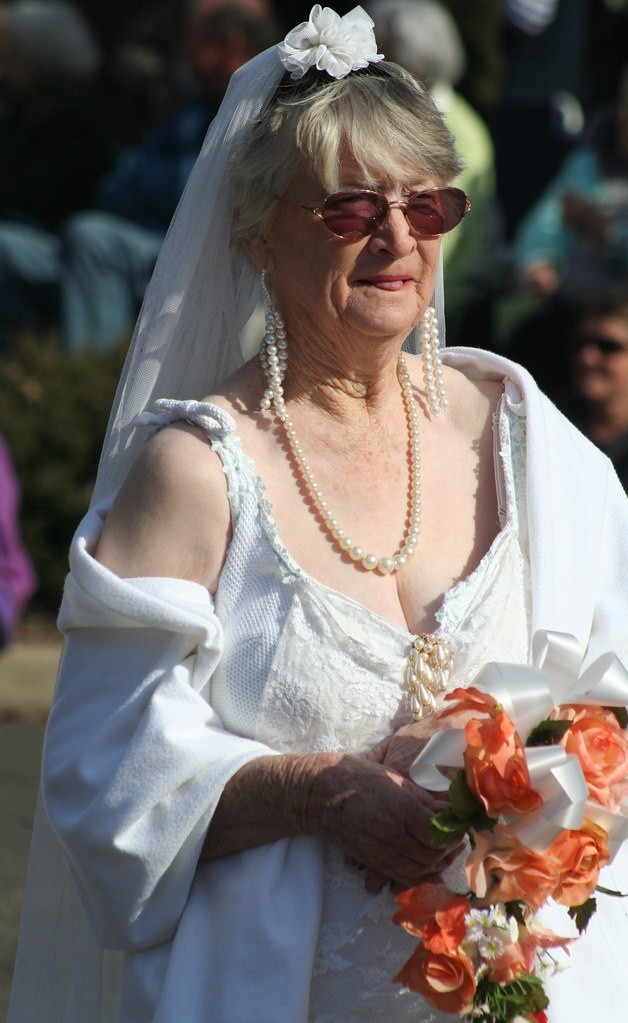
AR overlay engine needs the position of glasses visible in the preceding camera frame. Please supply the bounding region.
[277,187,472,239]
[571,335,628,356]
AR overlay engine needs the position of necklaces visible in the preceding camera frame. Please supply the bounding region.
[264,311,421,573]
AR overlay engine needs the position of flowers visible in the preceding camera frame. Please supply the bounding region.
[389,684,628,1023]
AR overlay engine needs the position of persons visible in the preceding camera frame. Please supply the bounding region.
[0,0,284,655]
[6,0,628,1023]
[370,0,628,486]
